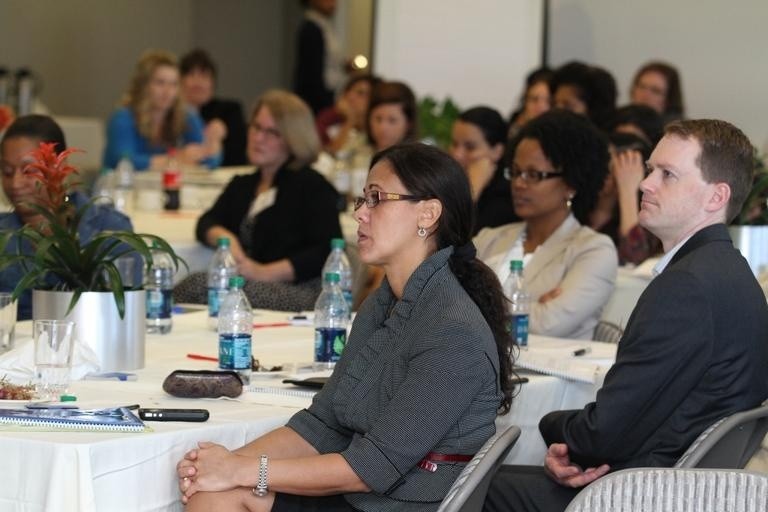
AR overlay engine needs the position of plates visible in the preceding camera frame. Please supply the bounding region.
[282,375,330,391]
[251,361,288,379]
[1,389,51,412]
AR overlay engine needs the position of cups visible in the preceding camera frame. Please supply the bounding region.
[31,317,75,398]
[1,292,20,350]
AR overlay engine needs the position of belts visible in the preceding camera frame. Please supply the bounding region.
[417,450,475,471]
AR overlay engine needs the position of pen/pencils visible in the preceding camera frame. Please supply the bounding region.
[573,347,593,357]
[37,395,76,402]
[371,0,545,124]
[252,323,292,328]
[187,354,219,362]
[125,405,140,410]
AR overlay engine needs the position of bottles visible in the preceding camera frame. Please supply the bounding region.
[142,235,174,335]
[114,147,135,214]
[159,145,182,211]
[312,271,353,372]
[97,165,116,206]
[206,237,237,318]
[322,236,355,296]
[500,258,531,352]
[216,274,254,388]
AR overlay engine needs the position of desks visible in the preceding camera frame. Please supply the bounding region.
[603,263,768,343]
[2,99,366,293]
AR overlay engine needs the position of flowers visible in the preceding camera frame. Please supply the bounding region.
[5,141,192,319]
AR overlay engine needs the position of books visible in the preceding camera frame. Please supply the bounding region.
[0,400,149,437]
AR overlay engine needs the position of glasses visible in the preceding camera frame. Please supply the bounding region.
[352,191,423,210]
[249,123,281,139]
[503,165,560,183]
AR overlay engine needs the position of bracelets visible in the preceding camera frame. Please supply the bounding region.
[251,452,270,501]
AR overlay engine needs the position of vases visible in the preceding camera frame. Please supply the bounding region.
[32,289,146,371]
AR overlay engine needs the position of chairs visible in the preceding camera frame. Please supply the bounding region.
[559,467,765,512]
[3,300,620,512]
[674,400,768,468]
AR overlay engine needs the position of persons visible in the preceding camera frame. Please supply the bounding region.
[173,91,343,311]
[291,2,352,113]
[105,44,249,171]
[0,114,146,324]
[316,75,417,172]
[449,59,684,265]
[176,139,520,512]
[482,118,767,512]
[473,108,618,340]
